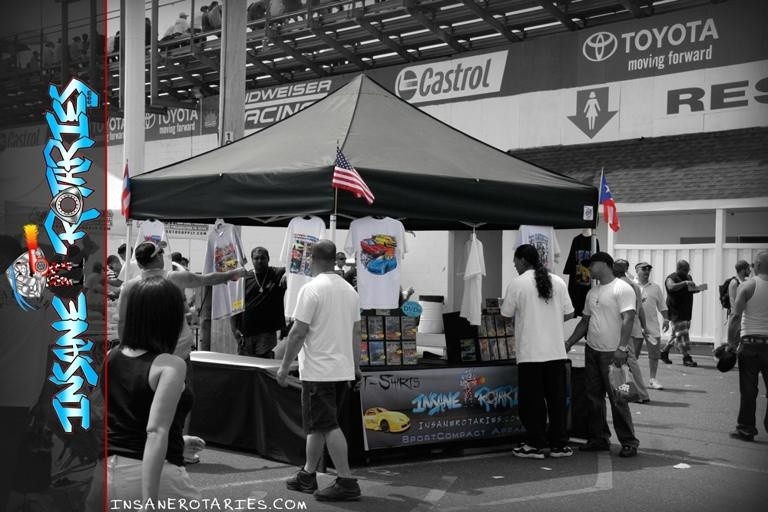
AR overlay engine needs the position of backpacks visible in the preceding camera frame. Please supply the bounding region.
[719,277,739,308]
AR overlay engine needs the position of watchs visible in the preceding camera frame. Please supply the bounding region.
[616,345,628,352]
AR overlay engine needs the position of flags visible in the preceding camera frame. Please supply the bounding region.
[120,161,133,224]
[597,169,621,233]
[333,146,376,206]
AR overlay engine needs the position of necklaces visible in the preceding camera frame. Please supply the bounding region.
[595,277,618,304]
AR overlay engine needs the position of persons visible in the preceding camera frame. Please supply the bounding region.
[563,251,640,458]
[611,256,754,404]
[116,240,251,464]
[276,237,365,502]
[77,241,189,343]
[499,243,575,459]
[227,246,288,358]
[333,251,350,274]
[86,273,204,511]
[724,248,767,442]
[0,233,94,510]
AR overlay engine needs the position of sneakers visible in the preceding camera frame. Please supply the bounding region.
[619,445,639,456]
[315,477,362,502]
[548,444,574,458]
[647,377,664,390]
[729,430,755,442]
[579,439,609,452]
[286,469,319,493]
[659,350,674,365]
[511,444,546,458]
[683,356,697,368]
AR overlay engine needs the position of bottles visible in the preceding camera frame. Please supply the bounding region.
[644,333,658,346]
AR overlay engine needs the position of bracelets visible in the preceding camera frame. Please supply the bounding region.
[564,340,571,350]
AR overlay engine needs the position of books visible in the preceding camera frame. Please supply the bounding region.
[354,314,419,366]
[459,295,516,361]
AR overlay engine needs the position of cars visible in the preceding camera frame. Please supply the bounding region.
[215,246,238,274]
[361,234,398,276]
[290,242,312,275]
[363,406,412,435]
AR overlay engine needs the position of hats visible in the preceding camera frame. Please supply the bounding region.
[716,352,737,372]
[580,251,614,268]
[734,259,753,273]
[634,261,652,269]
